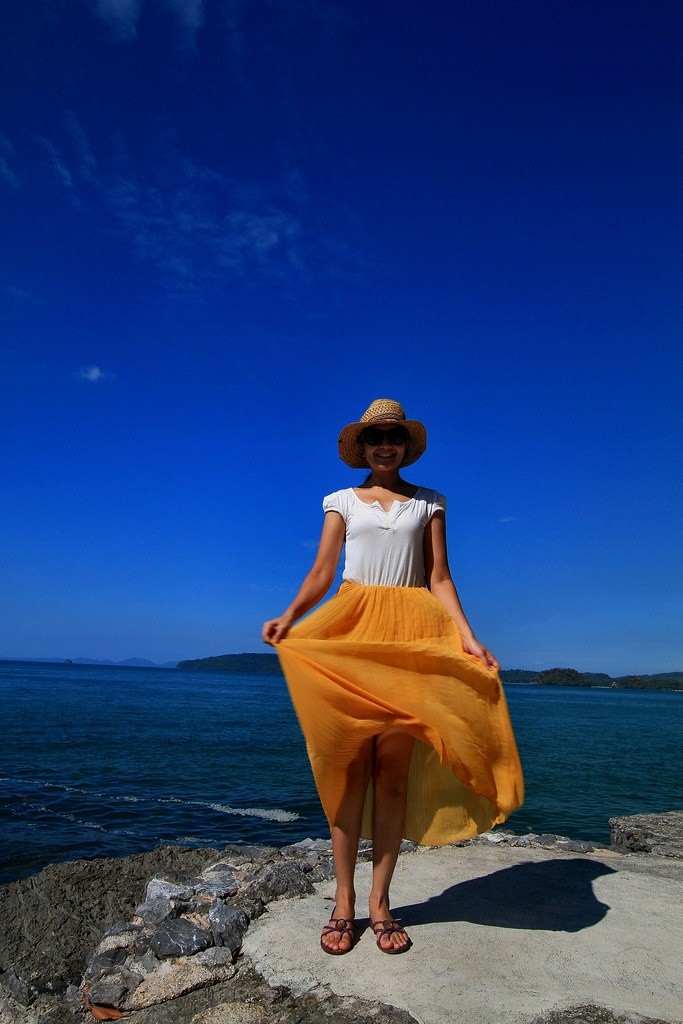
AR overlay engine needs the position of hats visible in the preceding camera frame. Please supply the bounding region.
[338,399,427,469]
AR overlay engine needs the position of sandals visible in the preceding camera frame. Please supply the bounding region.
[321,905,356,955]
[368,913,411,954]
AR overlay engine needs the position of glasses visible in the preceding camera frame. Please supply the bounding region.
[364,428,408,446]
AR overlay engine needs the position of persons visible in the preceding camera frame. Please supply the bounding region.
[262,399,501,955]
